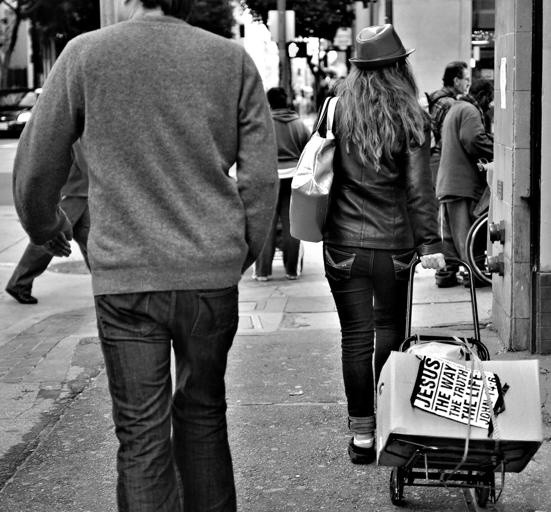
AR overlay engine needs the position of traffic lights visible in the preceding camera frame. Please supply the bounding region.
[282,40,306,58]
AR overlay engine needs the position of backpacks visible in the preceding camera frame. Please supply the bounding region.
[418,88,452,151]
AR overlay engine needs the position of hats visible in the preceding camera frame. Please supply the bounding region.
[345,21,417,70]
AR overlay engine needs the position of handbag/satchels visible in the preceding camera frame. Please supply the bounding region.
[284,94,341,244]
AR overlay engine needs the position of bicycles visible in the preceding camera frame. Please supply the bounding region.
[466,158,495,286]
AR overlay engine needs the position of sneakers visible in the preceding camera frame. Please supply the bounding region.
[347,432,378,465]
[286,273,298,280]
[252,272,269,281]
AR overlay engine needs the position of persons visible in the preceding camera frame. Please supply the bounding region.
[435,78,492,288]
[313,24,448,464]
[13,1,281,511]
[424,60,471,187]
[252,88,312,282]
[5,136,90,304]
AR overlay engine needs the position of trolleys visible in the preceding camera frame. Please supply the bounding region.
[384,255,499,508]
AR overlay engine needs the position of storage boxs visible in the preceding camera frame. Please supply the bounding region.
[373,352,544,475]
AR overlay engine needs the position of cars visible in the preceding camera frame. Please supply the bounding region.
[0,87,41,136]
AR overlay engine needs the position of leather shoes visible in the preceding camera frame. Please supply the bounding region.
[4,286,40,305]
